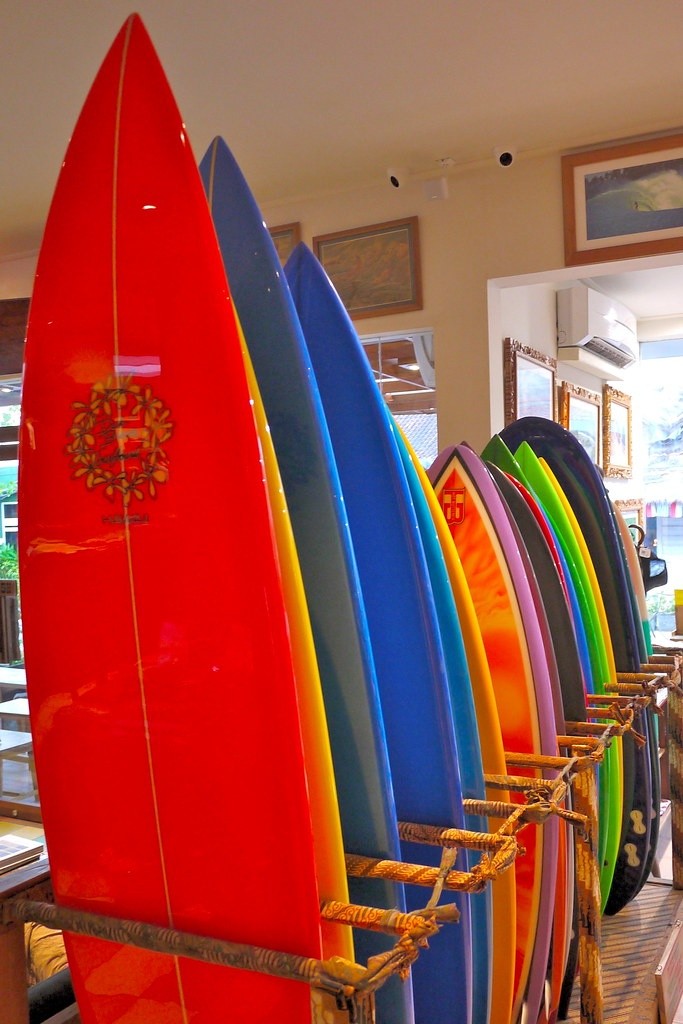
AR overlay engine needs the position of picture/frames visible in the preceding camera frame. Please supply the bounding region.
[603,383,633,480]
[559,134,683,267]
[505,338,557,426]
[614,498,643,549]
[313,215,424,322]
[560,380,603,469]
[267,221,301,269]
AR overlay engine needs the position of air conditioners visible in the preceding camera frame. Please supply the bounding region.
[556,287,638,369]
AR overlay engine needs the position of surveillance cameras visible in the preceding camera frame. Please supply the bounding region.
[387,167,400,188]
[494,146,515,168]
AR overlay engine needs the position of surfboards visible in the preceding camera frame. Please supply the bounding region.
[17,12,658,1024]
